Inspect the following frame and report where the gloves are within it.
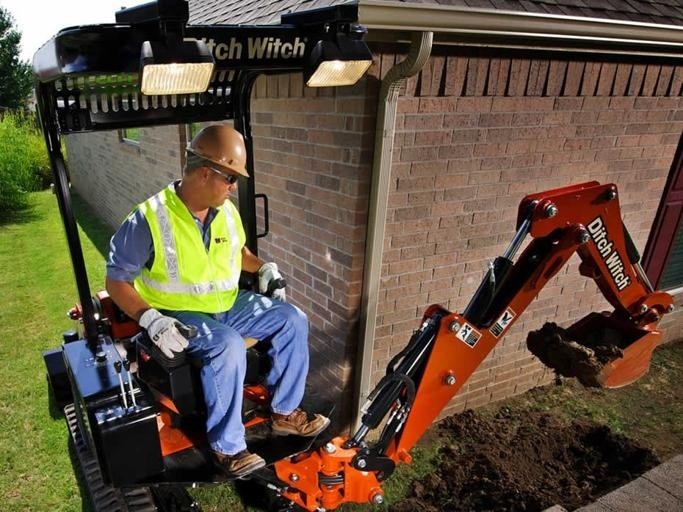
[139,308,190,359]
[258,263,286,303]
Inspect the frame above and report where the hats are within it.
[186,125,249,178]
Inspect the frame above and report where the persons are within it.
[104,124,331,477]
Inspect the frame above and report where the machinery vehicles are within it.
[25,2,677,511]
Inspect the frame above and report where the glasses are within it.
[210,165,237,184]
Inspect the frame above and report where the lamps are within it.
[113,1,373,97]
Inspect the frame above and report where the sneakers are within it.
[272,408,331,437]
[213,448,266,476]
